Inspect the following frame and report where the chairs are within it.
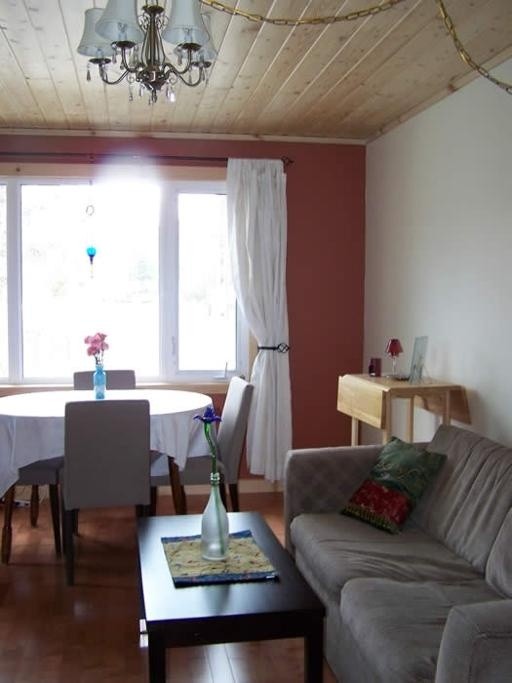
[15,465,61,554]
[72,370,144,536]
[150,376,254,516]
[63,400,150,587]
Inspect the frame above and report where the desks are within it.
[336,371,471,446]
[0,389,216,563]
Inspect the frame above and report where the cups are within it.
[369,358,381,376]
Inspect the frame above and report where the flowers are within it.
[84,332,109,366]
[193,407,225,556]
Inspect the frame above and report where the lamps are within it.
[77,0,219,103]
[385,338,404,380]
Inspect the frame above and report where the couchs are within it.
[284,424,512,683]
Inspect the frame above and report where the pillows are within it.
[339,436,447,535]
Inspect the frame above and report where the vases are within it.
[201,472,230,561]
[93,364,107,399]
